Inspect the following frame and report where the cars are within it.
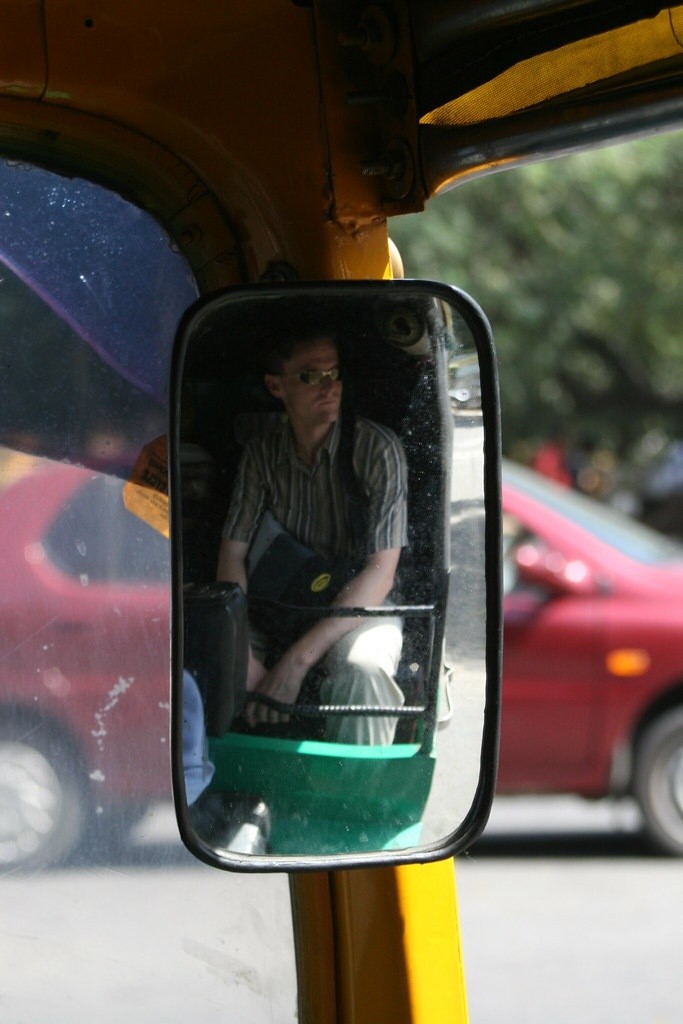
[0,445,683,872]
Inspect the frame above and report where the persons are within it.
[216,327,406,742]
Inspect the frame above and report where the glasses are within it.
[277,364,345,385]
